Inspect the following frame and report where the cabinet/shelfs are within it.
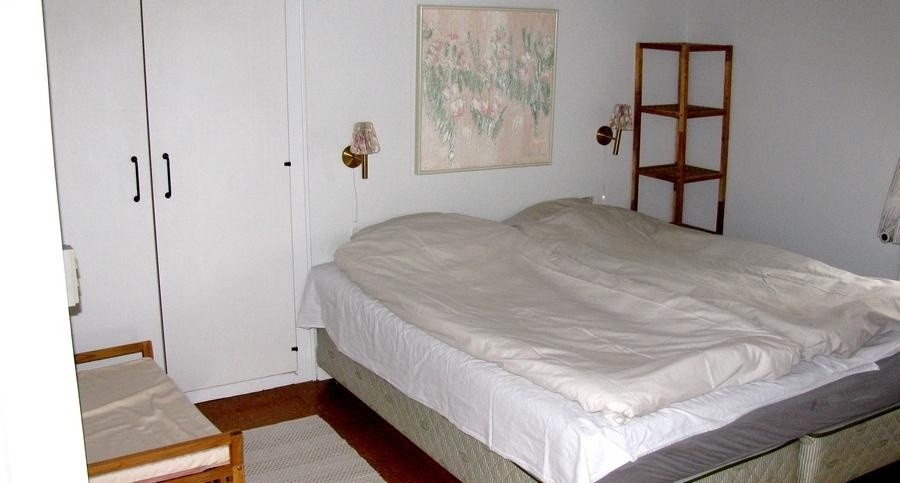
[631,41,733,234]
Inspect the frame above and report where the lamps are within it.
[342,120,379,179]
[596,104,632,155]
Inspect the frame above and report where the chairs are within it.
[74,341,245,483]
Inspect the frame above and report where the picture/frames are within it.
[416,3,559,176]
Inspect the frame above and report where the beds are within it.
[295,199,900,483]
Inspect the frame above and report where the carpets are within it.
[220,413,388,483]
